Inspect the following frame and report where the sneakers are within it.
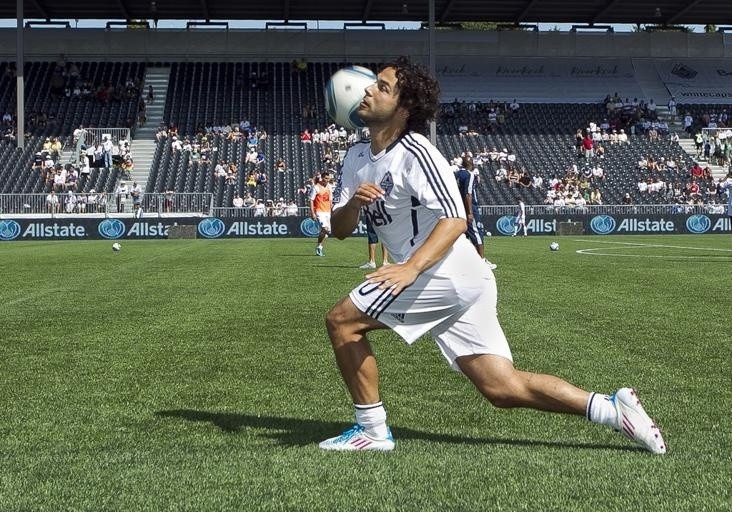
[360,261,377,269]
[316,245,324,255]
[318,425,397,451]
[614,387,667,455]
[483,258,498,271]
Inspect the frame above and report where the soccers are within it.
[485,231,491,237]
[549,241,559,252]
[112,243,121,251]
[322,64,379,132]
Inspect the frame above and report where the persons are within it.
[426,98,520,137]
[544,162,605,214]
[512,196,528,238]
[1,62,155,213]
[669,96,732,165]
[309,171,334,257]
[317,54,668,456]
[155,56,362,216]
[576,92,667,162]
[358,211,391,269]
[623,154,731,212]
[451,154,497,270]
[450,147,543,192]
